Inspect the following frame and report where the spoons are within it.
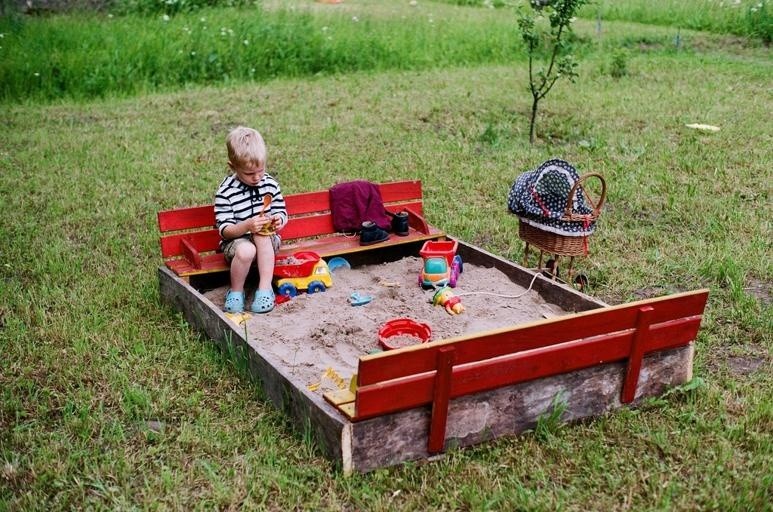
[259,194,272,218]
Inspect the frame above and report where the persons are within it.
[213,123,288,315]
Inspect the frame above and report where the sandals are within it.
[225,288,246,313]
[251,287,276,313]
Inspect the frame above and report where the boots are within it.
[360,220,389,244]
[392,212,409,236]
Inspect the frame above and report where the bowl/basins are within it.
[378,318,431,352]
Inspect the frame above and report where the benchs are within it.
[318,284,711,457]
[154,176,447,287]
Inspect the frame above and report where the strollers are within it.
[506,158,606,293]
